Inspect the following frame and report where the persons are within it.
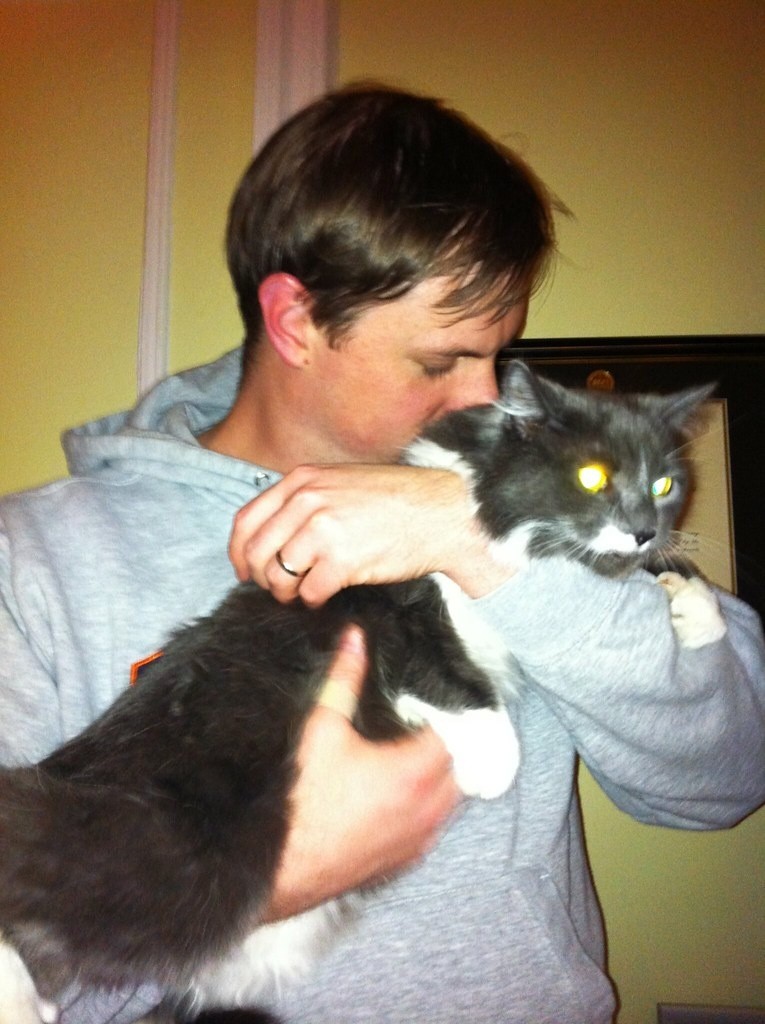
[0,82,765,1024]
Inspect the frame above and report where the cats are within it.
[0,358,719,1024]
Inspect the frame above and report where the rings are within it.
[317,680,359,721]
[276,550,306,578]
